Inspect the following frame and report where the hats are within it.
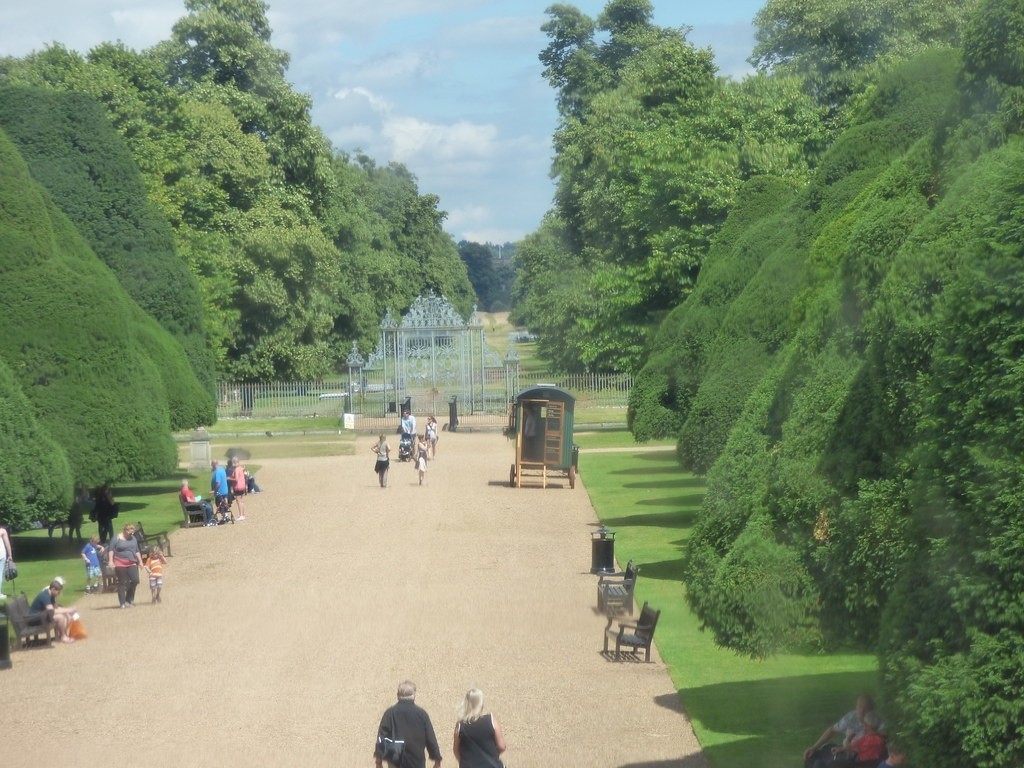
[54,577,66,586]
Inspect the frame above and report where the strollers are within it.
[399,433,415,462]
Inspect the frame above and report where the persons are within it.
[399,409,416,455]
[362,377,368,398]
[47,486,88,545]
[372,681,443,768]
[81,535,104,593]
[425,416,437,459]
[108,522,144,608]
[805,695,909,768]
[0,527,13,599]
[370,433,391,486]
[180,479,217,526]
[209,456,263,524]
[415,434,428,486]
[144,546,168,604]
[452,688,506,768]
[90,484,115,543]
[525,405,537,462]
[222,381,240,404]
[27,576,76,644]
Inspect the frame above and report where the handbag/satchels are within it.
[415,460,420,469]
[90,509,98,522]
[66,615,87,640]
[6,559,17,581]
[384,709,406,765]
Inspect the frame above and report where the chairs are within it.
[179,494,211,528]
[97,543,139,593]
[135,521,173,559]
[604,601,661,663]
[597,559,638,616]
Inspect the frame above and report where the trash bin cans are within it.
[589,525,617,575]
[563,446,579,473]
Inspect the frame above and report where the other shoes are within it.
[236,516,245,521]
[87,582,162,609]
[208,522,218,526]
[62,635,75,643]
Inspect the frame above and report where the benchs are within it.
[3,591,51,649]
